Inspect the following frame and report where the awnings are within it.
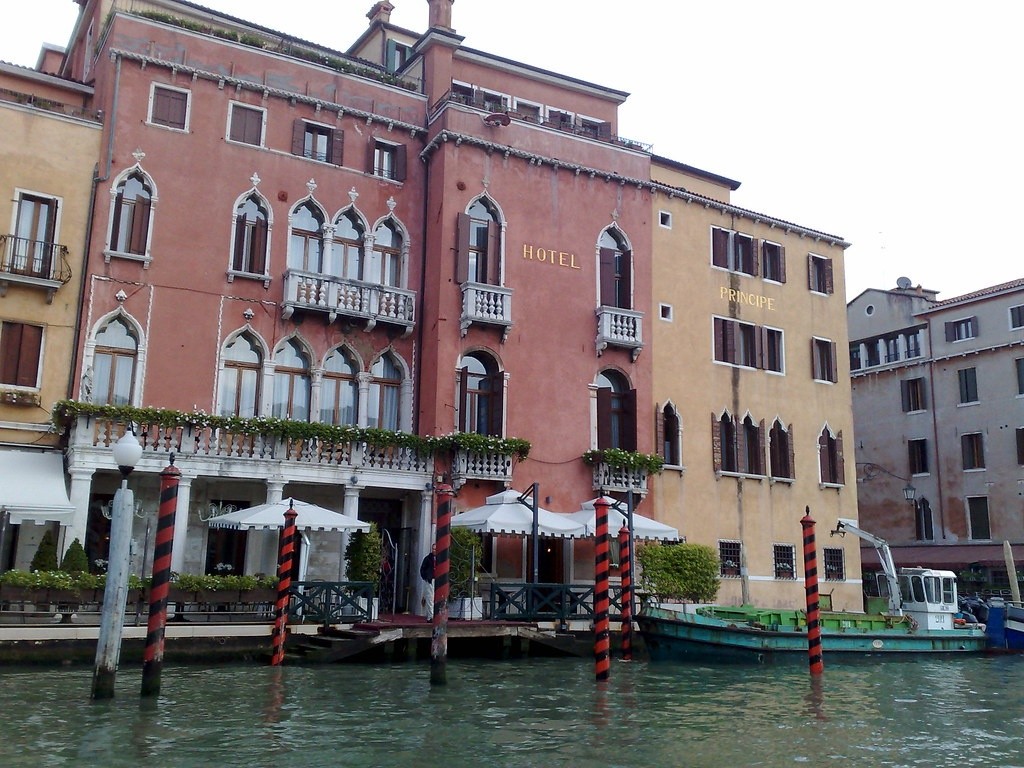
[0,449,73,528]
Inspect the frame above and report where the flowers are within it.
[582,448,666,477]
[452,92,468,101]
[145,572,199,593]
[439,430,532,463]
[723,560,738,568]
[0,569,50,590]
[50,571,98,591]
[48,399,192,436]
[97,574,144,591]
[359,425,439,459]
[239,576,280,592]
[777,563,792,572]
[193,403,281,441]
[282,414,360,446]
[130,9,418,90]
[826,564,838,575]
[199,573,241,592]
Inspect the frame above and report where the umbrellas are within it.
[208,478,680,617]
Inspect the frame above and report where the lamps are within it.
[902,483,916,507]
[100,499,148,519]
[197,501,243,531]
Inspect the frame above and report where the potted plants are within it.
[342,520,386,620]
[488,101,505,113]
[29,528,59,612]
[448,525,484,620]
[560,122,576,133]
[471,96,486,110]
[520,112,539,124]
[577,126,598,139]
[539,119,560,129]
[55,538,89,624]
[626,142,643,151]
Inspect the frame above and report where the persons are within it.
[957,592,989,624]
[420,543,436,622]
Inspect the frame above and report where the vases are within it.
[196,591,240,602]
[396,80,404,88]
[240,588,276,601]
[95,590,140,603]
[369,74,376,80]
[452,97,466,104]
[200,27,213,35]
[609,140,625,146]
[505,111,523,120]
[143,590,196,602]
[406,83,414,91]
[830,574,838,579]
[47,590,95,602]
[726,567,737,575]
[595,136,608,142]
[778,571,793,578]
[0,584,48,603]
